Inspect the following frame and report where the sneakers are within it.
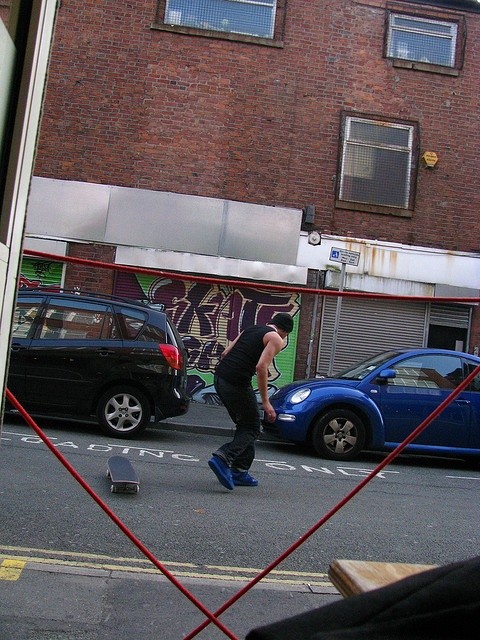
[208,454,235,490]
[230,468,259,486]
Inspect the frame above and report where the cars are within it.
[263,324,480,464]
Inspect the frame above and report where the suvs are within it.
[0,287,190,436]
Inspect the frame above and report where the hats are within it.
[273,312,294,333]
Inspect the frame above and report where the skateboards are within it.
[105,455,140,494]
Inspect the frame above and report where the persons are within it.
[208,313,294,490]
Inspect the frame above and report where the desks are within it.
[329,557,440,598]
